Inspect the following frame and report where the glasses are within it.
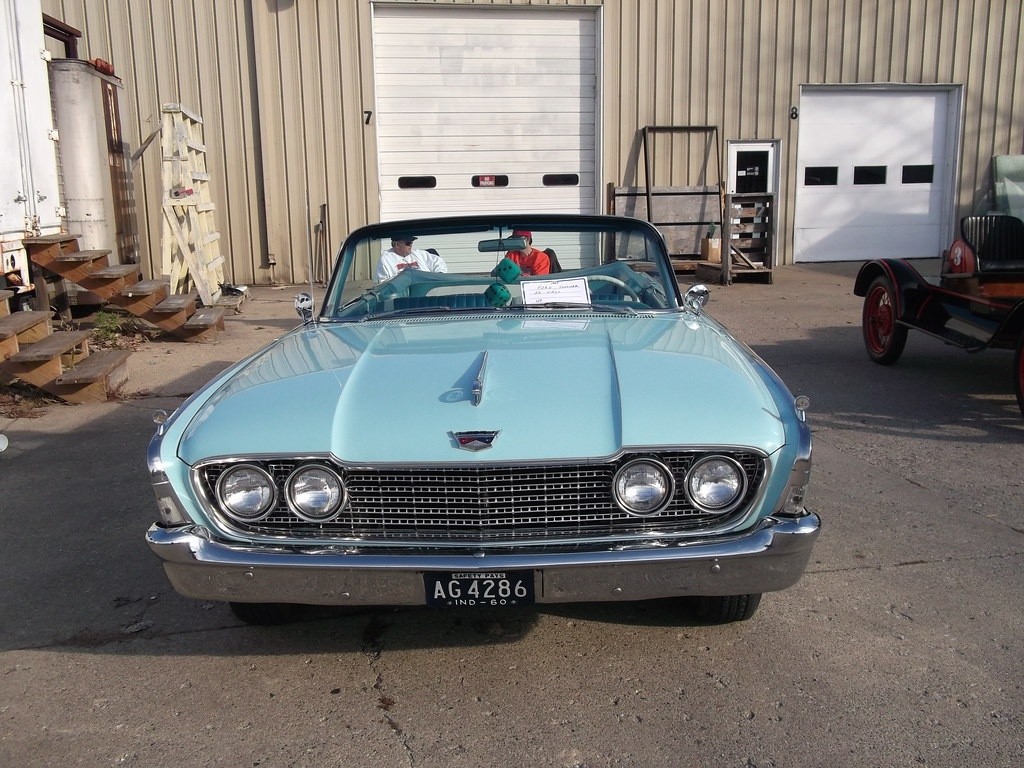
[398,241,413,246]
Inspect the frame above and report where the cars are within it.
[144,212,820,625]
[852,214,1024,417]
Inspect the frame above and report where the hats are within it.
[391,236,418,241]
[507,230,531,239]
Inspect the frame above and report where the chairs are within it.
[960,216,1024,299]
[384,293,491,312]
[590,300,652,310]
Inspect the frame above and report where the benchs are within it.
[409,279,624,308]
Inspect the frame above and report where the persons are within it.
[374,236,447,284]
[505,230,550,276]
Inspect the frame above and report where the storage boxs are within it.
[701,237,722,262]
